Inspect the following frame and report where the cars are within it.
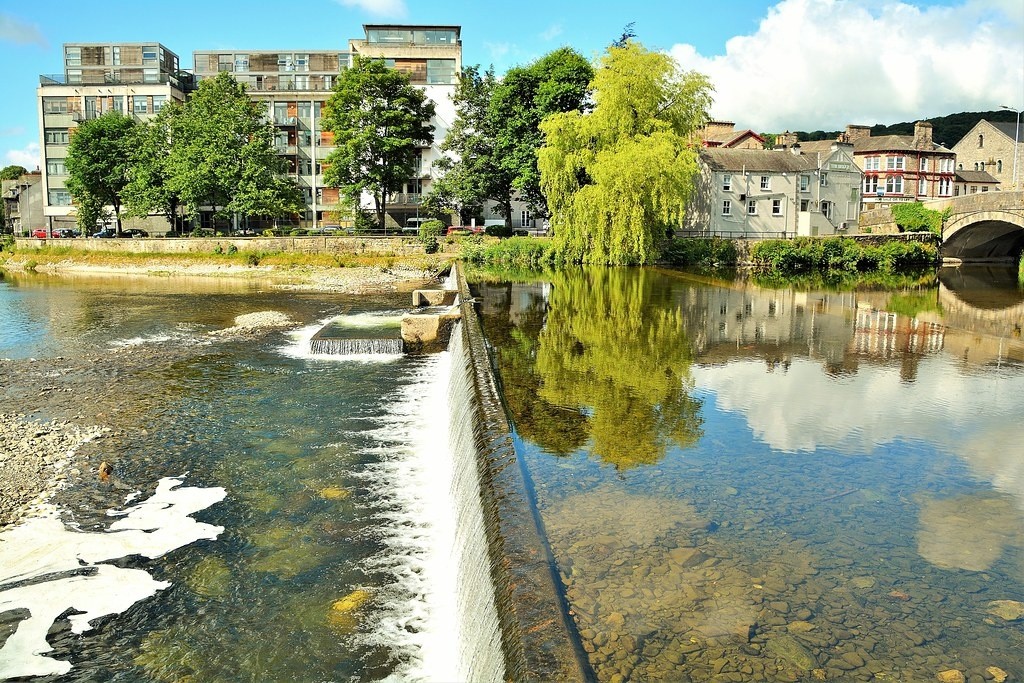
[448,226,479,234]
[32,229,82,239]
[165,231,180,237]
[118,228,149,238]
[92,228,116,238]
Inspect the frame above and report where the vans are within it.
[402,217,438,236]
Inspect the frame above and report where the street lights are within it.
[999,104,1021,186]
